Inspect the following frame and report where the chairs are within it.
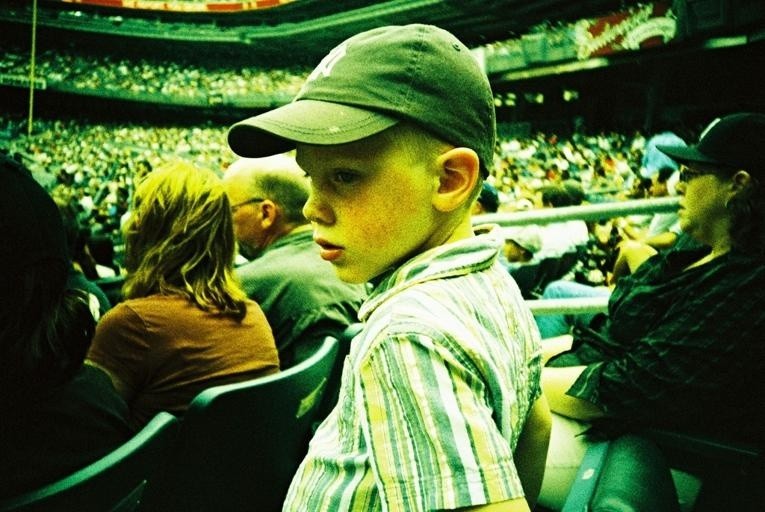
[1,243,683,510]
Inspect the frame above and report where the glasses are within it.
[677,166,721,184]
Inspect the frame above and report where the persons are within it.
[1,8,764,512]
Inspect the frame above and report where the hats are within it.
[653,110,765,168]
[224,21,500,182]
